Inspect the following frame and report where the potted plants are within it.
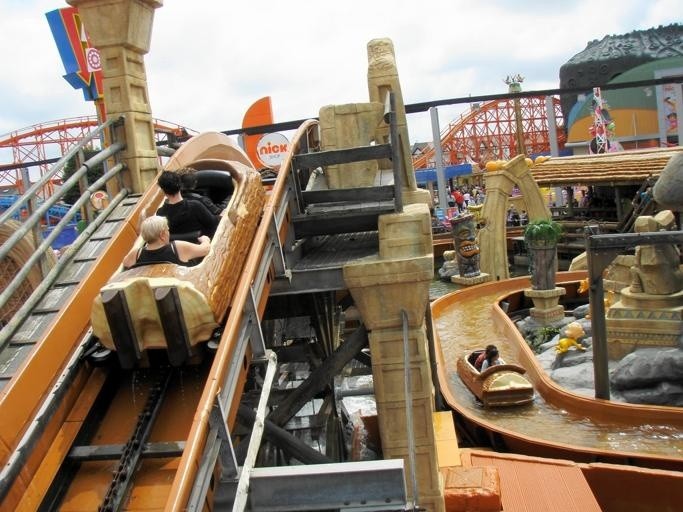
[523,215,563,291]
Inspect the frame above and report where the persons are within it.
[562,186,656,222]
[505,204,528,226]
[429,182,486,234]
[123,166,231,271]
[663,97,677,132]
[472,344,504,374]
